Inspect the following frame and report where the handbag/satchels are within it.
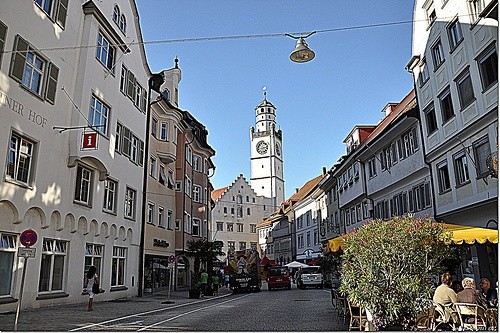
[92,276,100,294]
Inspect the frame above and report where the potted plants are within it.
[186,240,225,299]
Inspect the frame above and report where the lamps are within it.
[285,31,317,63]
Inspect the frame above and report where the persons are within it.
[225,273,229,289]
[432,272,499,310]
[212,274,219,296]
[86,266,99,312]
[201,269,208,295]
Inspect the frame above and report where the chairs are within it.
[332,289,499,332]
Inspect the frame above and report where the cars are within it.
[295,264,323,290]
[266,266,293,291]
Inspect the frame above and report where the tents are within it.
[327,220,499,252]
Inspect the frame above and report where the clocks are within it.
[257,143,268,153]
